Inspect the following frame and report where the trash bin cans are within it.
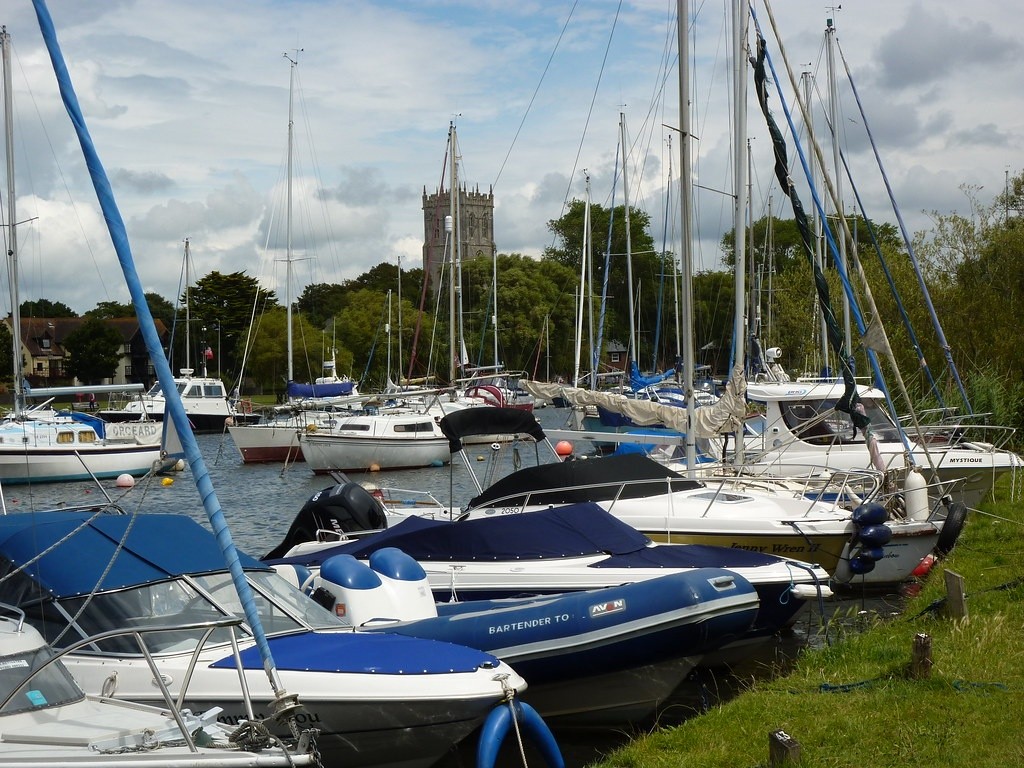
[75,393,85,402]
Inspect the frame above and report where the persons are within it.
[109,392,117,408]
[233,386,241,399]
[88,392,96,411]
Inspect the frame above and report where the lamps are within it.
[766,347,782,362]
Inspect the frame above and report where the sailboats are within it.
[0,0,1024,768]
